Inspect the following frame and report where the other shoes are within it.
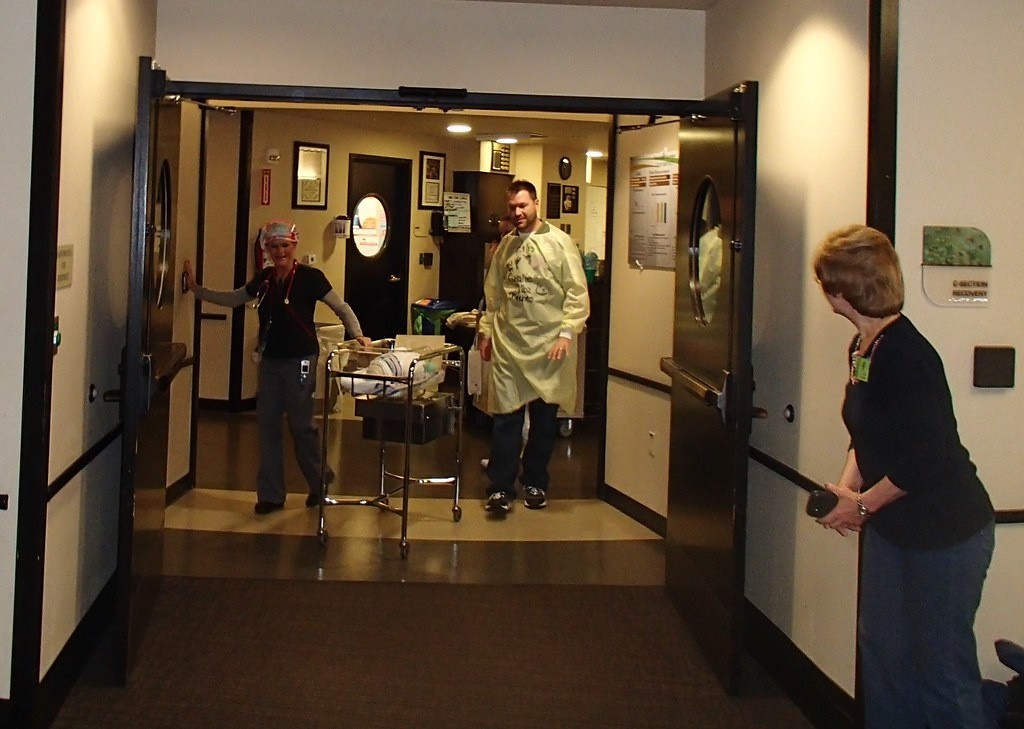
[480,459,489,468]
[255,501,284,514]
[520,444,527,460]
[305,473,335,507]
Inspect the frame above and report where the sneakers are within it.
[485,490,514,510]
[522,485,546,507]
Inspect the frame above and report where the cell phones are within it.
[806,490,838,518]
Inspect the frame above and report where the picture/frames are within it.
[418,151,446,210]
[292,140,327,207]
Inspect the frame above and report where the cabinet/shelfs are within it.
[452,170,514,242]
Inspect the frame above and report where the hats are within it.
[260,219,299,250]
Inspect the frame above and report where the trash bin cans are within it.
[411,298,461,386]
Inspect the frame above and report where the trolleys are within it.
[446,295,574,439]
[316,342,466,562]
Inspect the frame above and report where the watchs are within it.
[856,494,873,518]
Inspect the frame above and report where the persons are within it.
[695,206,723,323]
[184,219,372,513]
[811,227,997,729]
[477,179,591,514]
